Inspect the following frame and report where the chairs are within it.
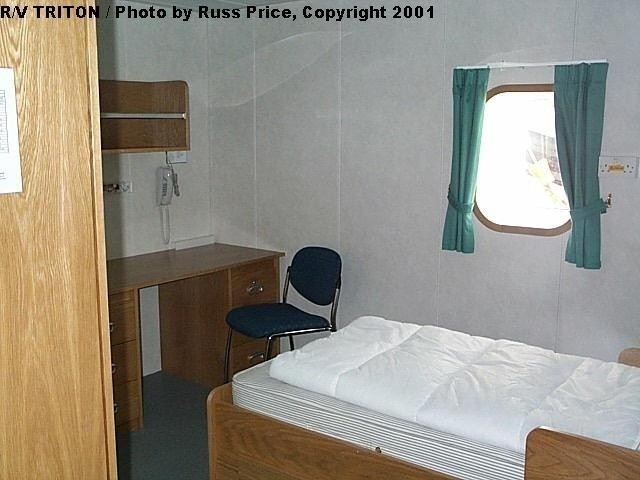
[224,246,343,384]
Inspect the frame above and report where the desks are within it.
[106,240,286,438]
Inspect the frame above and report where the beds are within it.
[206,315,640,480]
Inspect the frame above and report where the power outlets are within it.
[166,151,188,164]
[597,156,639,178]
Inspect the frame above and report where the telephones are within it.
[156,166,174,206]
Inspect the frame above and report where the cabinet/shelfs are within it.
[99,79,190,155]
[0,2,118,480]
